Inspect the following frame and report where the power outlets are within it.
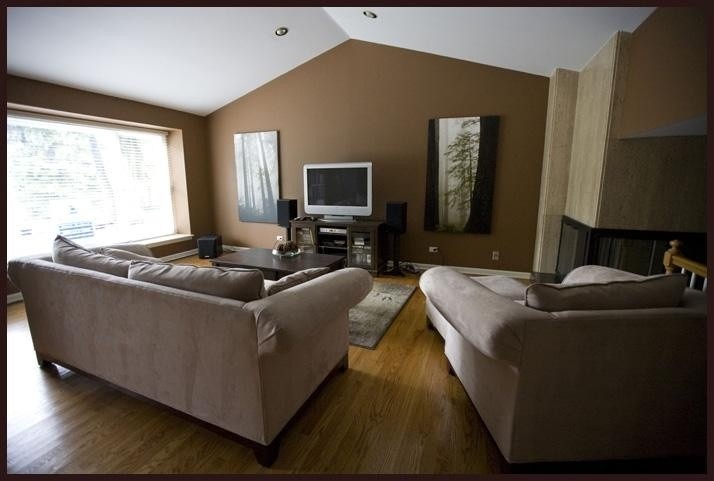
[428,245,440,254]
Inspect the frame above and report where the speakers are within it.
[277,199,297,227]
[386,202,407,235]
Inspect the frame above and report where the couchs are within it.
[8,241,374,468]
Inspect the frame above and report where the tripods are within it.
[384,235,405,277]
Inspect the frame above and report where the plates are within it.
[272,249,301,258]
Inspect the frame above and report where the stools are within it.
[421,270,526,332]
[196,233,224,257]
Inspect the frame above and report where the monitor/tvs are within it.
[303,162,373,222]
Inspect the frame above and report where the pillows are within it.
[49,233,133,278]
[266,264,330,299]
[126,259,263,301]
[523,271,689,314]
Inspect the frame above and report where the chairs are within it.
[417,258,709,470]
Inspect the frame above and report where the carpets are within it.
[345,278,418,349]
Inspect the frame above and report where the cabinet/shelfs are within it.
[287,217,387,277]
